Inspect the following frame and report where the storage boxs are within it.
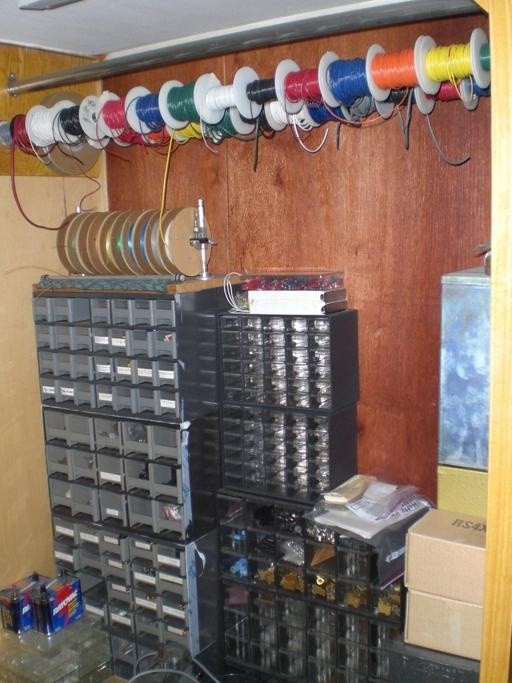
[437,266,491,472]
[437,464,488,520]
[404,589,484,661]
[404,507,487,606]
